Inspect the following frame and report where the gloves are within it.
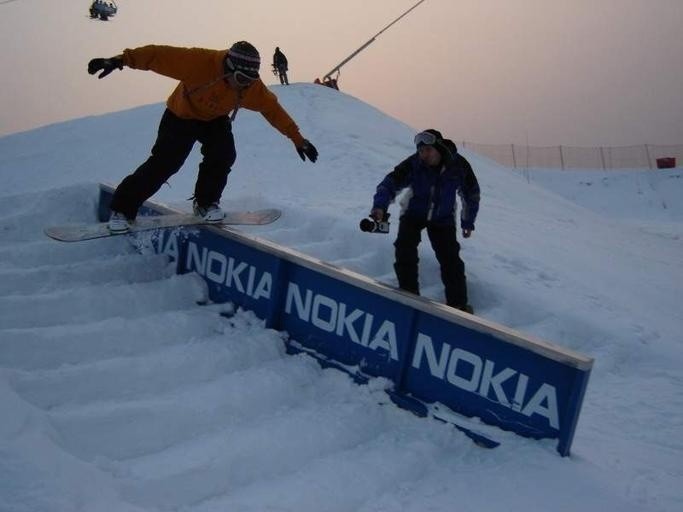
[296,139,318,162]
[88,56,124,79]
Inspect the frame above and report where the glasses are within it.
[225,57,260,88]
[415,132,443,145]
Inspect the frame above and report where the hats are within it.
[416,130,443,154]
[225,41,260,78]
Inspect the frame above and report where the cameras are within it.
[360,212,390,233]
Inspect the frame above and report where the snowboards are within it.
[44,209,281,242]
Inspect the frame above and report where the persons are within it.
[370,128,479,312]
[89,0,118,20]
[270,44,288,87]
[83,41,320,235]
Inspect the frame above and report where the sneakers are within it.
[108,210,129,234]
[193,198,224,220]
